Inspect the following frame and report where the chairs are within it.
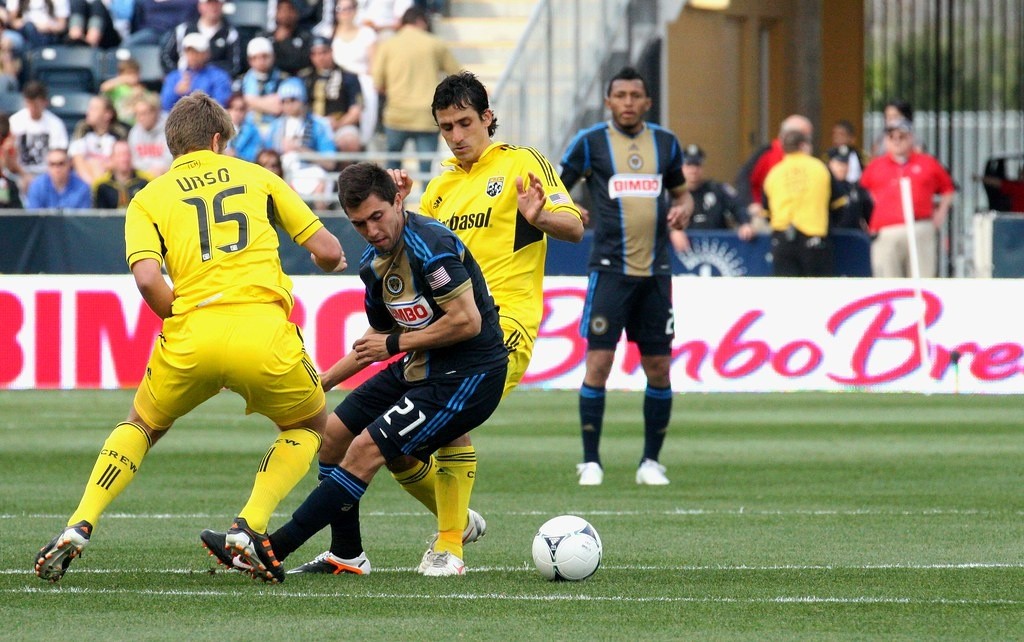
[2,42,173,151]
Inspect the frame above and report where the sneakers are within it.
[226,517,285,584]
[636,465,670,486]
[33,519,93,584]
[201,529,234,570]
[416,508,487,574]
[578,462,603,485]
[424,552,467,575]
[301,552,371,575]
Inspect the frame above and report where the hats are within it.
[278,76,309,104]
[678,149,705,166]
[246,37,272,55]
[182,32,210,52]
[827,146,858,162]
[308,37,330,47]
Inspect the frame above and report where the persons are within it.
[667,97,957,278]
[0,0,468,208]
[382,66,585,579]
[198,161,512,584]
[34,91,349,585]
[557,64,696,487]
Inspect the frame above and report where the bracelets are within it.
[385,332,403,357]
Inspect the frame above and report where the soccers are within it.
[531,513,603,582]
